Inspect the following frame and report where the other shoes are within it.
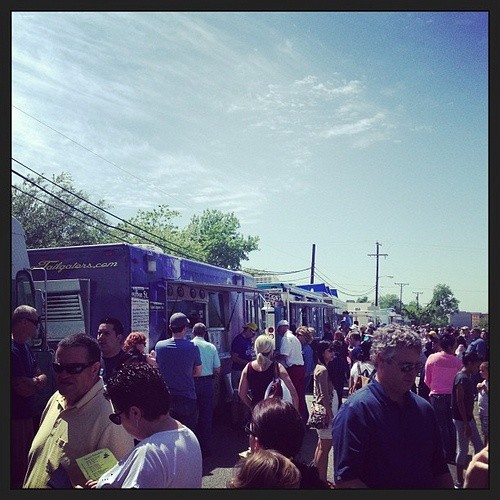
[455,480,465,489]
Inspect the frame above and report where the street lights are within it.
[375,276,394,306]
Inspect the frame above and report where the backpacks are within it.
[348,362,377,396]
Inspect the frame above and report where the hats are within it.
[276,320,288,331]
[242,323,259,331]
[429,331,436,337]
[170,312,190,328]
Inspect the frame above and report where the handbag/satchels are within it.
[269,362,283,401]
[308,402,330,429]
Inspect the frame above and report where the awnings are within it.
[289,301,342,324]
[166,279,269,330]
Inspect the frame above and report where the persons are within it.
[190,322,221,432]
[424,333,461,464]
[275,320,304,417]
[231,322,258,402]
[155,312,202,433]
[452,351,484,489]
[331,323,455,488]
[244,398,331,489]
[226,449,301,489]
[323,311,487,410]
[84,360,203,489]
[22,333,134,488]
[476,362,489,445]
[237,334,299,410]
[122,332,157,369]
[296,326,315,395]
[97,318,133,386]
[10,305,48,489]
[313,341,338,488]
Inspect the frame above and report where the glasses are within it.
[244,423,255,437]
[251,330,256,335]
[23,318,38,326]
[52,363,93,375]
[389,358,420,372]
[109,410,125,425]
[140,342,146,346]
[327,348,336,353]
